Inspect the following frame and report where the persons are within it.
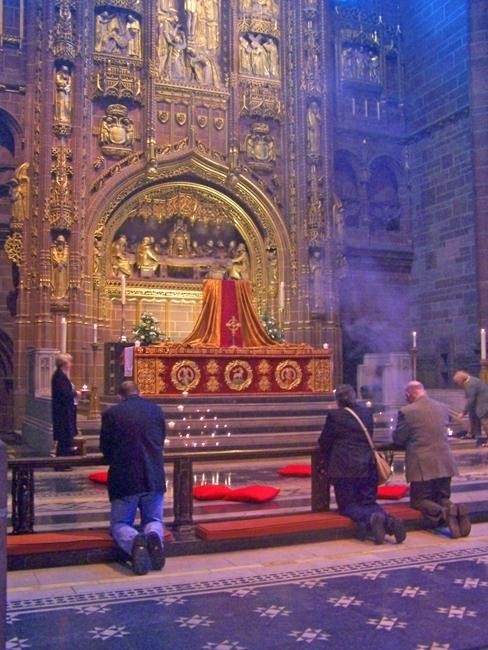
[317,383,407,545]
[98,380,168,576]
[51,234,69,299]
[110,235,135,279]
[392,379,472,539]
[136,236,161,271]
[229,243,250,280]
[50,352,83,472]
[54,65,72,122]
[453,369,488,447]
[168,218,192,258]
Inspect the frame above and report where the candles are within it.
[120,275,126,304]
[92,324,98,343]
[278,282,285,308]
[479,329,486,360]
[58,318,67,354]
[411,331,417,348]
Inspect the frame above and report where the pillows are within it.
[277,462,312,477]
[377,484,409,499]
[227,480,279,503]
[193,484,233,500]
[87,470,108,484]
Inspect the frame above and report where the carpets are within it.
[2,538,487,650]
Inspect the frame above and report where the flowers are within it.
[261,312,286,343]
[130,310,168,344]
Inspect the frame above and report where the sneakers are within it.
[372,512,386,545]
[457,503,471,537]
[147,531,165,571]
[131,533,149,576]
[443,502,462,538]
[391,516,406,543]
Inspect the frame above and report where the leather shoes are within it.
[460,434,475,439]
[482,442,487,447]
[55,466,73,471]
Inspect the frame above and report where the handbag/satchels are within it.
[375,457,393,485]
